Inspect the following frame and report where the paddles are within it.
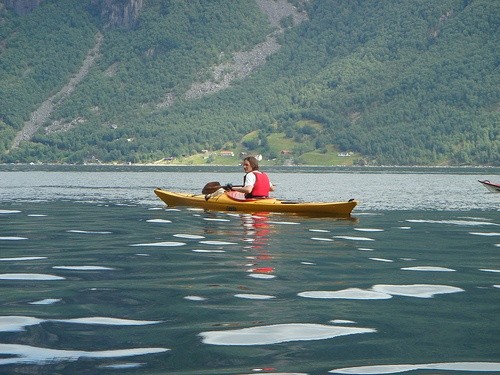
[202,181,276,195]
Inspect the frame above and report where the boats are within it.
[477,179,500,192]
[153,189,357,215]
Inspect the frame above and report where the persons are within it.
[224,156,275,199]
